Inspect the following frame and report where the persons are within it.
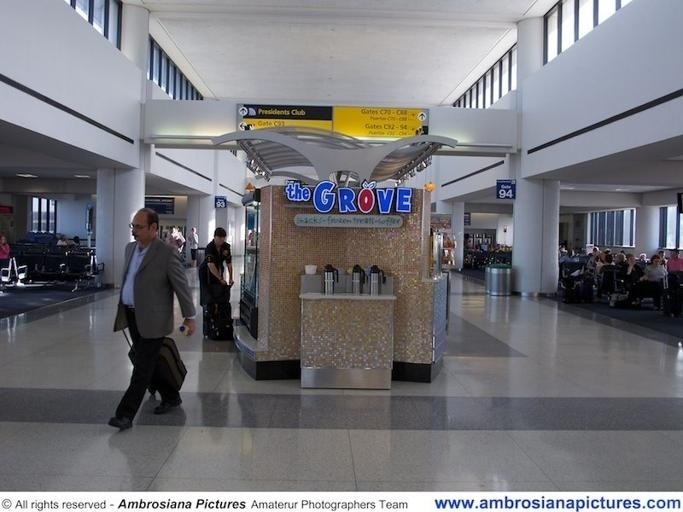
[197,228,233,338]
[68,236,79,247]
[165,226,198,268]
[56,234,68,246]
[104,206,196,430]
[464,238,683,313]
[1,234,10,259]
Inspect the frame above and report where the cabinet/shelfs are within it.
[298,292,396,391]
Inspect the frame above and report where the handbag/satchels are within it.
[200,279,236,342]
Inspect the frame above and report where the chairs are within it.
[559,244,683,316]
[0,231,97,293]
[463,243,512,268]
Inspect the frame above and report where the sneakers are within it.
[151,394,184,416]
[107,413,134,430]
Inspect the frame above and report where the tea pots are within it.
[370,264,387,295]
[324,263,338,295]
[351,264,366,295]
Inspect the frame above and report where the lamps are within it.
[394,142,441,189]
[239,142,272,182]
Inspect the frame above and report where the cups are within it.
[304,264,317,275]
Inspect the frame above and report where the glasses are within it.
[128,223,153,231]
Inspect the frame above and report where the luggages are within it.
[123,334,188,396]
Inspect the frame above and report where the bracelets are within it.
[218,278,222,281]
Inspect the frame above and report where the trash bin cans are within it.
[485,264,512,296]
[196,248,206,267]
